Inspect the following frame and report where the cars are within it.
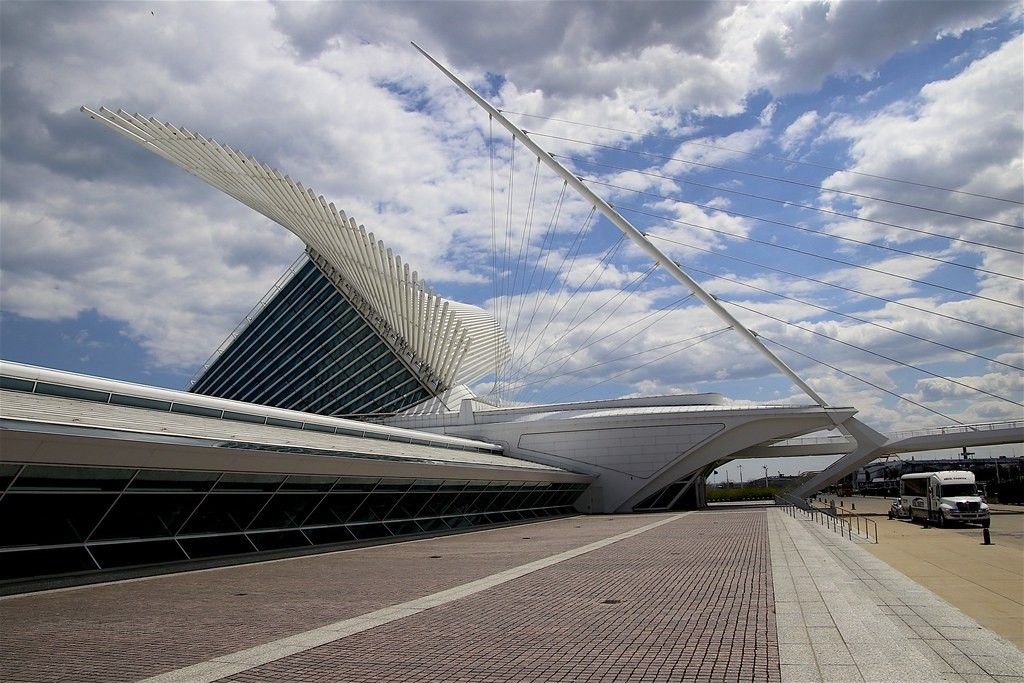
[890,498,908,519]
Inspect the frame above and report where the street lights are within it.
[737,464,744,488]
[762,465,769,487]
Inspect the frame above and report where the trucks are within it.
[901,471,991,529]
